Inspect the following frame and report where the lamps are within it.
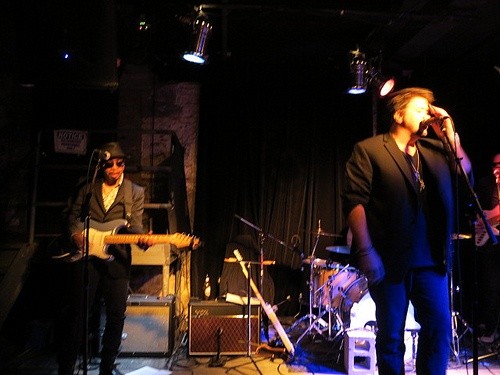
[182,4,214,64]
[347,44,397,98]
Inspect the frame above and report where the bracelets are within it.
[355,245,374,259]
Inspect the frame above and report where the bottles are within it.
[202,274,211,301]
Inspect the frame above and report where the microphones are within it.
[292,243,305,259]
[419,116,446,132]
[95,148,111,161]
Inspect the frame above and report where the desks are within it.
[128,240,181,322]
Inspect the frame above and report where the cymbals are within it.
[325,245,351,254]
[449,232,473,240]
[304,229,344,238]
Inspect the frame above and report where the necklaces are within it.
[397,144,425,192]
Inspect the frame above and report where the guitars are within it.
[58,215,203,266]
[475,204,500,246]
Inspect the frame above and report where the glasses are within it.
[103,159,125,168]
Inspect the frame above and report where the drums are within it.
[310,263,358,306]
[299,257,341,308]
[340,275,420,365]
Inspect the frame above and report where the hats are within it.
[101,141,125,161]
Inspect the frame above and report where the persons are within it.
[59,142,153,375]
[340,88,471,375]
[471,152,500,351]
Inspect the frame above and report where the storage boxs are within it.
[189,297,262,356]
[100,294,175,357]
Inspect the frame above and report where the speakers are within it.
[188,299,261,355]
[98,293,175,357]
